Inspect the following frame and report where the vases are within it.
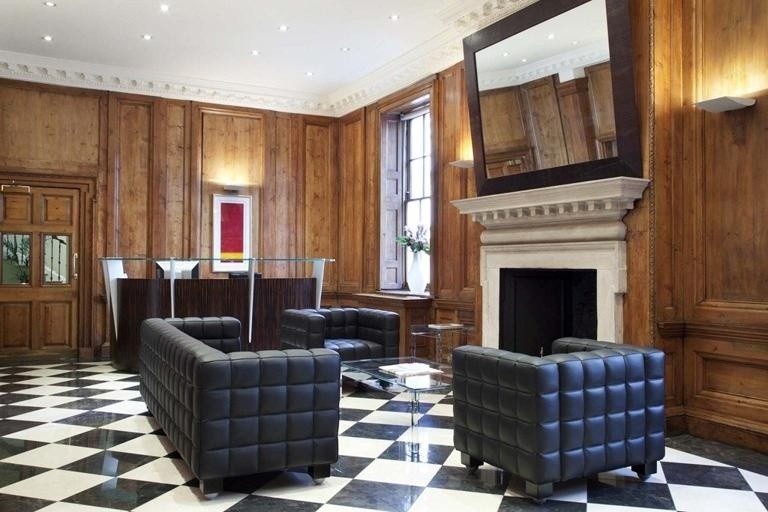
[405,251,427,293]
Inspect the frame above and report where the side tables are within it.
[409,323,470,386]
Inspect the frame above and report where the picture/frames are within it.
[211,193,253,273]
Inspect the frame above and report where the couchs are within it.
[137,315,340,500]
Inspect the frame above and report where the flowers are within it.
[394,225,430,256]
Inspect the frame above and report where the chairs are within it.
[452,336,666,505]
[276,305,400,391]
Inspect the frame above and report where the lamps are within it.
[692,95,757,115]
[223,185,241,193]
[449,159,475,170]
